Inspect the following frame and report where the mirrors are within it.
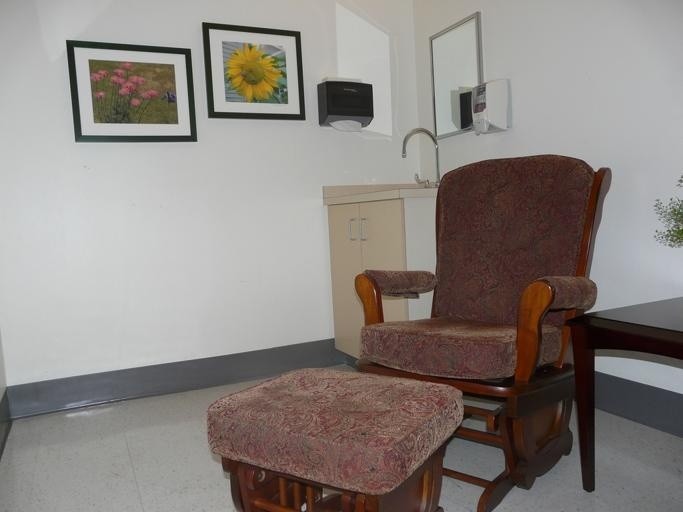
[427,12,484,142]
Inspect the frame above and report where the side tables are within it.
[563,295,681,492]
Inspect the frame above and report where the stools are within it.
[205,365,464,511]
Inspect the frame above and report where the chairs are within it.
[354,154,612,512]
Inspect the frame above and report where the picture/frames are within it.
[64,19,307,144]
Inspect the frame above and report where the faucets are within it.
[401,127,440,188]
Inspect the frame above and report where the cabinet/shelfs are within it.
[327,199,437,361]
